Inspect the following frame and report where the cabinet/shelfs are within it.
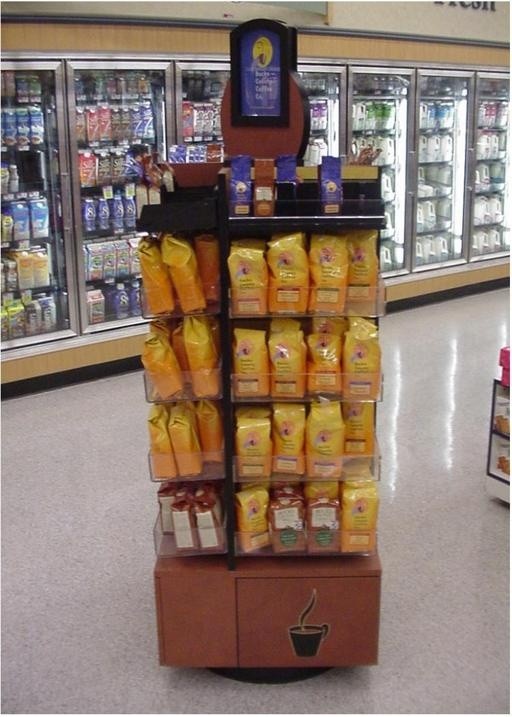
[140,162,382,684]
[483,378,510,504]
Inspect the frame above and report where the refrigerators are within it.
[470,70,512,263]
[343,62,416,277]
[74,62,174,335]
[0,58,81,357]
[413,66,475,272]
[292,64,348,168]
[170,62,235,165]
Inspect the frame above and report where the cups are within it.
[288,621,329,656]
